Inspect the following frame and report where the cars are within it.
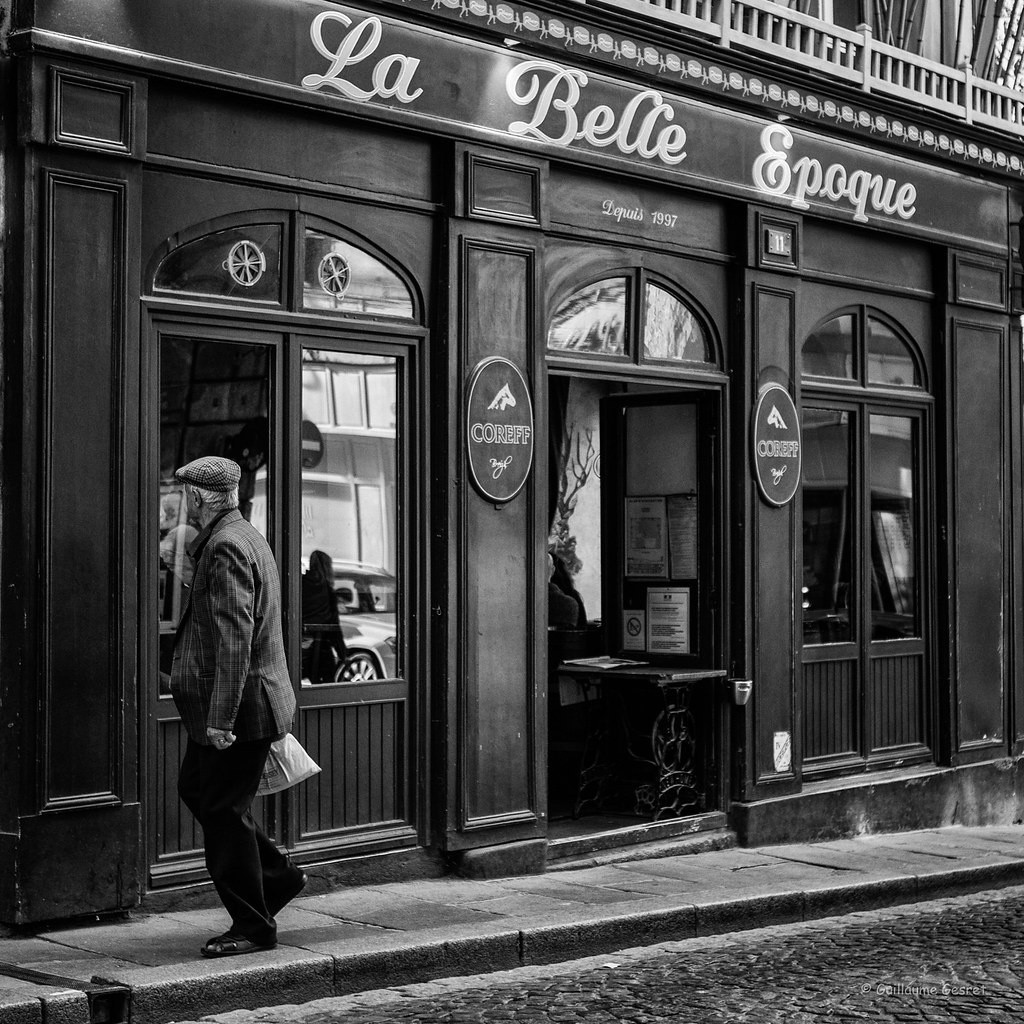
[299,559,398,686]
[802,606,914,644]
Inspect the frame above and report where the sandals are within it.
[199,930,277,954]
[265,864,306,916]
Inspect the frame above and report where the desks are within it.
[556,658,726,825]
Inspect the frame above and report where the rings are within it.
[219,739,225,742]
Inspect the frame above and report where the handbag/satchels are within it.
[255,731,322,796]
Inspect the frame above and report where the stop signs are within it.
[300,420,323,468]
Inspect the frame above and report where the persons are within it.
[547,551,588,625]
[303,549,350,685]
[160,493,201,620]
[174,456,309,956]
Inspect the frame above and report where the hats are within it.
[174,455,241,492]
